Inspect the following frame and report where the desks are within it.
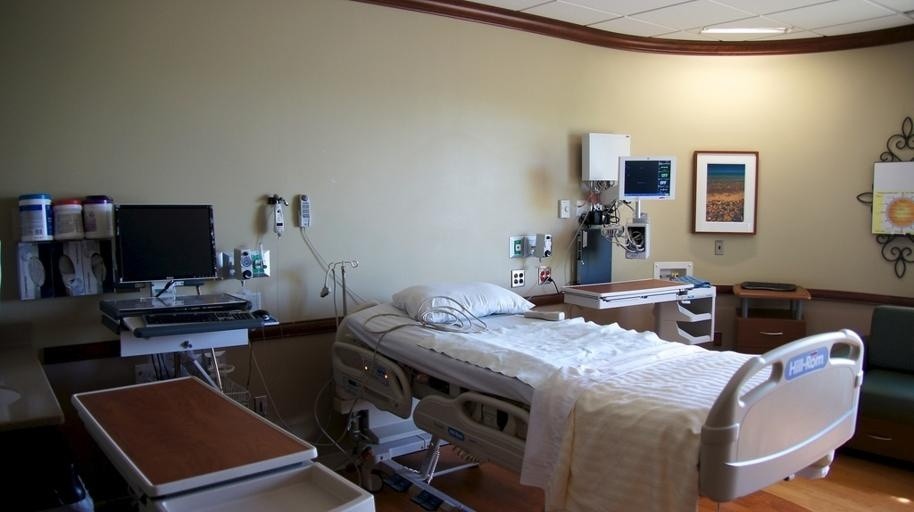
[553,272,695,313]
[93,292,277,397]
[66,371,378,512]
[0,298,348,512]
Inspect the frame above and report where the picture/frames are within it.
[687,146,760,239]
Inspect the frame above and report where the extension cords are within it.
[297,194,311,228]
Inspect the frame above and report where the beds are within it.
[322,270,873,512]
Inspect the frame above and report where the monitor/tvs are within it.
[113,204,218,298]
[619,156,677,200]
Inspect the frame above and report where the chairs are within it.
[833,299,913,474]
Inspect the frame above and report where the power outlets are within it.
[507,235,553,289]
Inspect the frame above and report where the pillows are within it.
[389,274,537,335]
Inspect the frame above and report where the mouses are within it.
[253,310,271,320]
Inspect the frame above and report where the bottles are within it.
[54,198,84,240]
[85,194,112,240]
[19,194,51,242]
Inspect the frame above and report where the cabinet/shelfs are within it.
[654,280,719,347]
[729,275,812,358]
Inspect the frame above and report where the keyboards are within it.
[143,312,256,328]
